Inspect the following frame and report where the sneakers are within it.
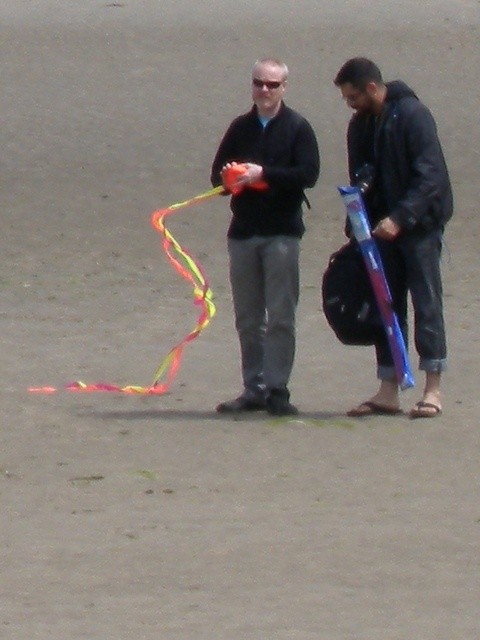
[265,392,298,415]
[217,395,264,412]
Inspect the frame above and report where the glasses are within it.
[342,90,365,101]
[252,79,285,90]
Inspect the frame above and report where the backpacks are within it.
[322,239,384,345]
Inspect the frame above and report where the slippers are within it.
[410,401,442,418]
[347,400,404,419]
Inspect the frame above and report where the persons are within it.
[210,57,321,415]
[324,57,454,419]
